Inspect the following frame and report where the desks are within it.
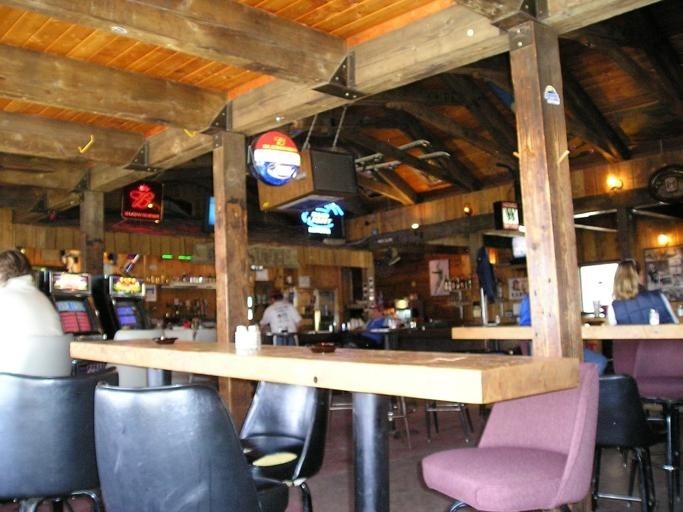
[69,325,582,511]
[451,327,681,376]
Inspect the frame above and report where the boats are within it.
[256,150,359,213]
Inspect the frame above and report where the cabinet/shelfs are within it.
[444,274,473,291]
[471,300,483,327]
[234,325,262,353]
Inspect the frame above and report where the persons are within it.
[606,256,681,327]
[648,260,664,290]
[0,250,72,379]
[255,289,299,347]
[344,305,403,350]
[517,279,530,326]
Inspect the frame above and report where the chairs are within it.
[95,379,290,510]
[2,369,118,512]
[421,363,599,507]
[236,380,325,512]
[584,338,682,508]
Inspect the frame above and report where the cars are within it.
[593,299,600,319]
[649,307,661,328]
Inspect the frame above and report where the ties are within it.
[115,300,146,331]
[505,221,526,265]
[55,298,96,334]
[292,202,347,242]
[201,194,215,235]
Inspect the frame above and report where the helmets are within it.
[309,342,337,353]
[151,336,178,345]
